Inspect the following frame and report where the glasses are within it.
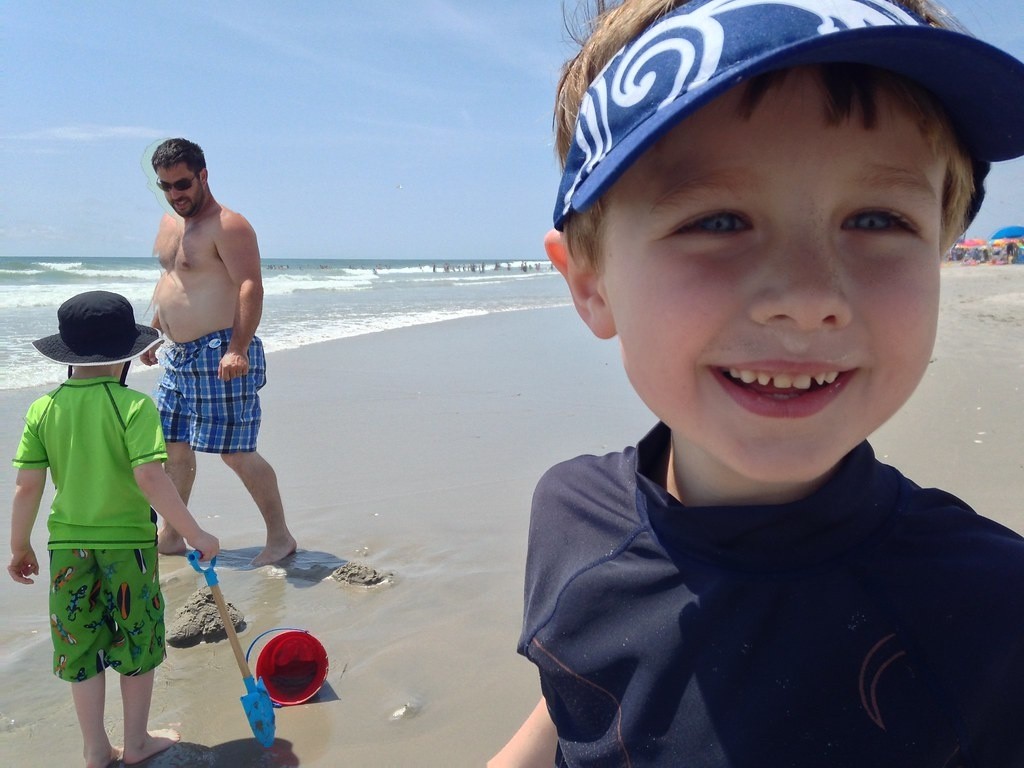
[156,174,199,191]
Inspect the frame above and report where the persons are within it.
[488,2,1023,768]
[139,138,297,567]
[5,291,221,767]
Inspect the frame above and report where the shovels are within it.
[188,550,276,746]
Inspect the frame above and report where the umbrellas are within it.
[955,226,1024,249]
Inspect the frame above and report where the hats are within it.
[32,291,164,366]
[554,0,1024,231]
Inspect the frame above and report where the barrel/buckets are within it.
[246,627,329,708]
